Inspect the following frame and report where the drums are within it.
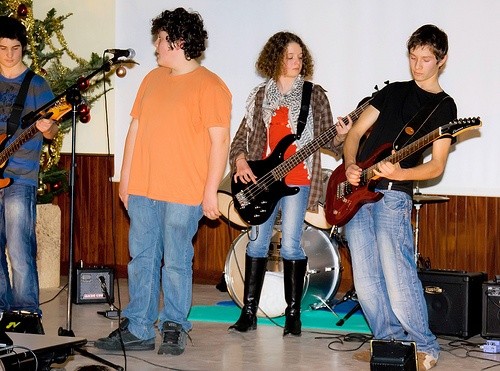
[221,224,343,317]
[216,171,254,228]
[304,168,333,230]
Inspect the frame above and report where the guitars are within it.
[0,93,85,190]
[216,80,391,230]
[323,116,482,227]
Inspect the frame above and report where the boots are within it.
[228,254,269,334]
[282,255,308,337]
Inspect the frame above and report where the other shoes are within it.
[352,349,371,362]
[417,351,439,371]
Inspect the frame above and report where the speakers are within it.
[416,269,499,340]
[71,268,115,304]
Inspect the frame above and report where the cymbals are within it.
[411,194,450,205]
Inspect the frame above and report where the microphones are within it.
[105,48,135,59]
[98,276,106,286]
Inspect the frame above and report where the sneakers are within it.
[0,311,45,334]
[158,321,192,355]
[94,318,155,350]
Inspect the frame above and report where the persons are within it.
[0,17,61,320]
[228,31,353,337]
[343,24,456,371]
[92,8,232,357]
